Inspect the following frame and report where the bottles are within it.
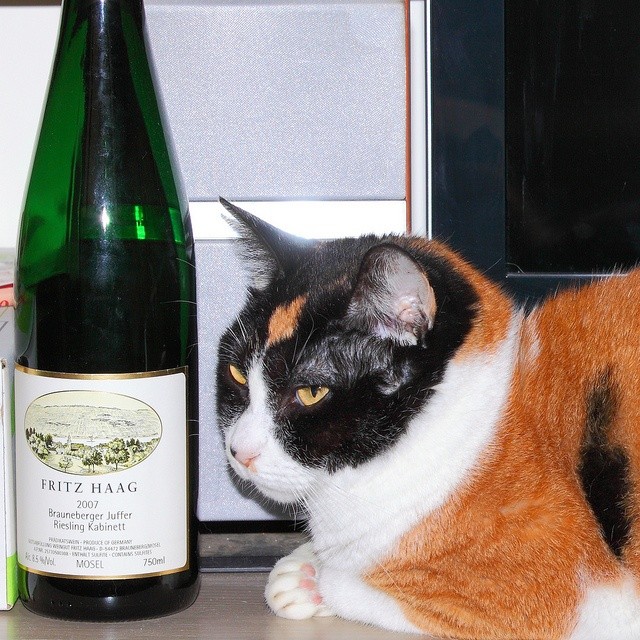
[14,0,200,623]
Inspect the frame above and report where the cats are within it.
[178,193,640,640]
